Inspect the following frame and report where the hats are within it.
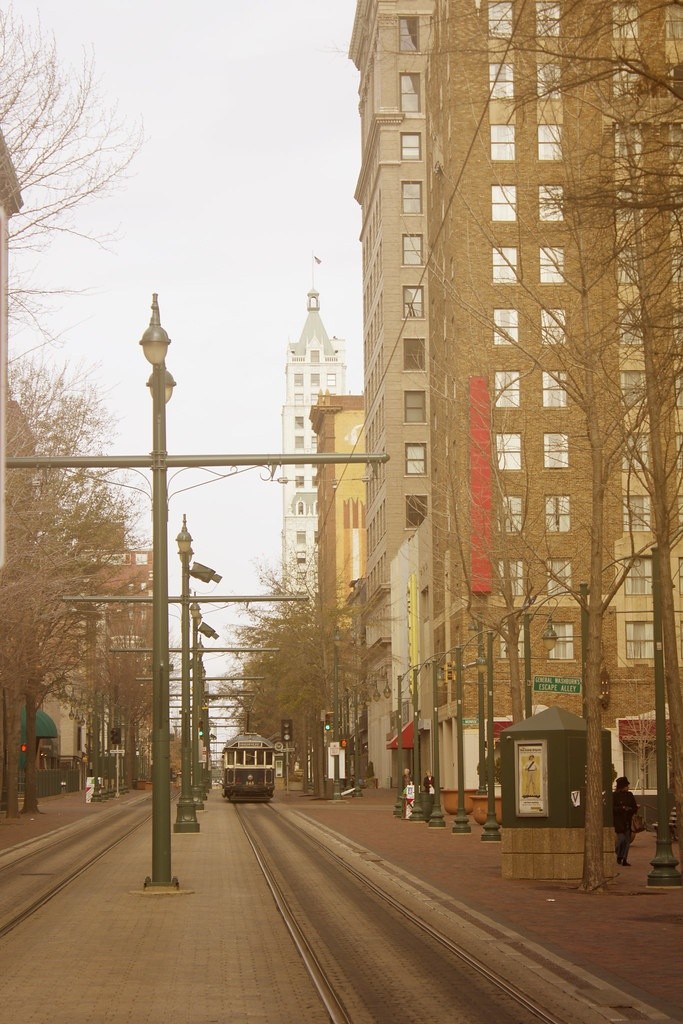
[616,777,630,790]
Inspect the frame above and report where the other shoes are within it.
[622,862,632,866]
[617,857,621,864]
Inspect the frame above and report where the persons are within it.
[423,769,435,793]
[612,776,638,866]
[350,775,355,788]
[402,768,413,792]
[523,755,538,795]
[343,776,348,787]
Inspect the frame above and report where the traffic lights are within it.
[281,719,293,742]
[340,739,347,747]
[324,713,330,731]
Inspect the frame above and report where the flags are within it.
[314,257,321,264]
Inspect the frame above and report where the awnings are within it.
[485,721,513,742]
[386,721,414,750]
[35,710,58,738]
[619,720,671,742]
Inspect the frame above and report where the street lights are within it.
[523,582,595,716]
[138,291,183,893]
[454,642,487,836]
[483,612,559,837]
[333,624,343,801]
[342,678,392,804]
[188,590,213,810]
[174,511,201,836]
[393,643,463,828]
[581,554,675,725]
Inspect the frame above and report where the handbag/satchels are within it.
[429,784,434,794]
[630,811,645,832]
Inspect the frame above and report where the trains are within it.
[222,731,276,804]
